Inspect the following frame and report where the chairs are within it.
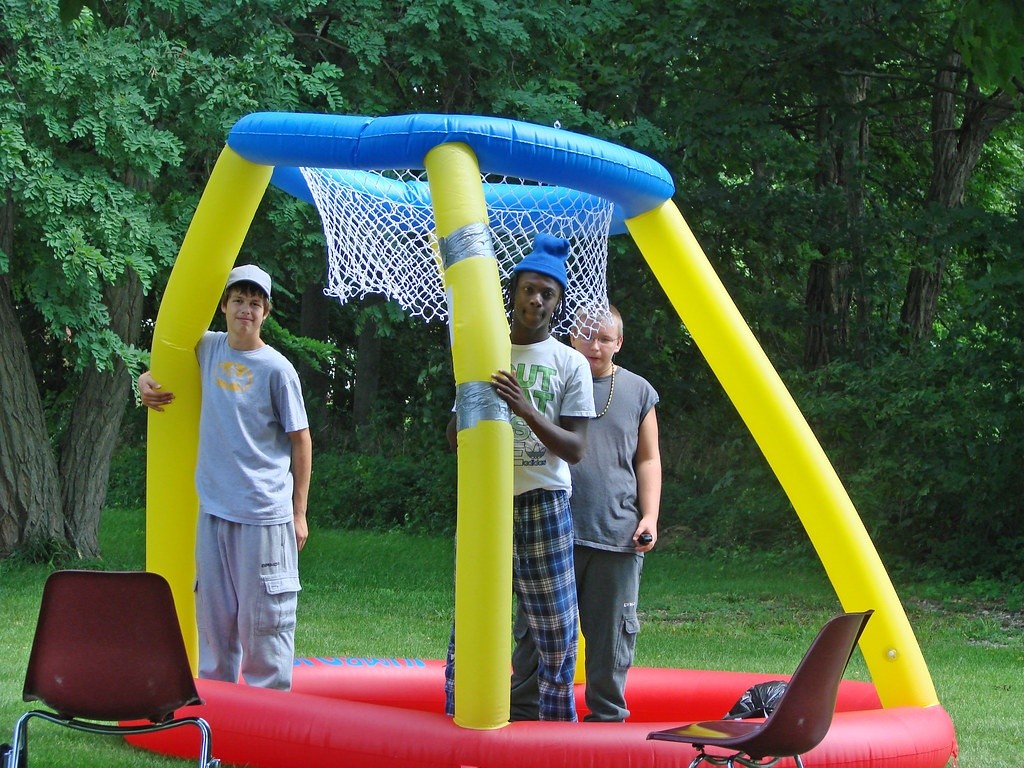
[6,568,222,768]
[648,609,877,768]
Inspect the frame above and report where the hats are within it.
[226,265,271,301]
[513,233,570,290]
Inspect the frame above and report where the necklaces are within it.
[591,362,614,418]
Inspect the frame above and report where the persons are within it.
[139,265,312,691]
[507,304,662,722]
[446,237,595,724]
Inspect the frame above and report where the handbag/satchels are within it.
[725,680,788,731]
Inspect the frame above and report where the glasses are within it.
[577,334,620,344]
